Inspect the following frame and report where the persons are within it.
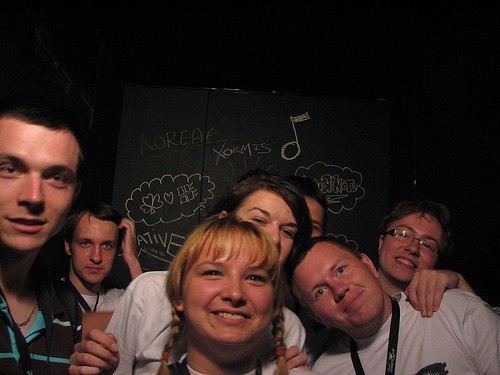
[68,176,475,375]
[158,211,314,375]
[0,113,83,375]
[288,236,500,375]
[65,205,142,312]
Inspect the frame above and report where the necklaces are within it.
[17,303,37,327]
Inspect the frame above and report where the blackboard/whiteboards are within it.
[110,82,392,286]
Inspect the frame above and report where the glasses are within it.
[384,228,441,256]
